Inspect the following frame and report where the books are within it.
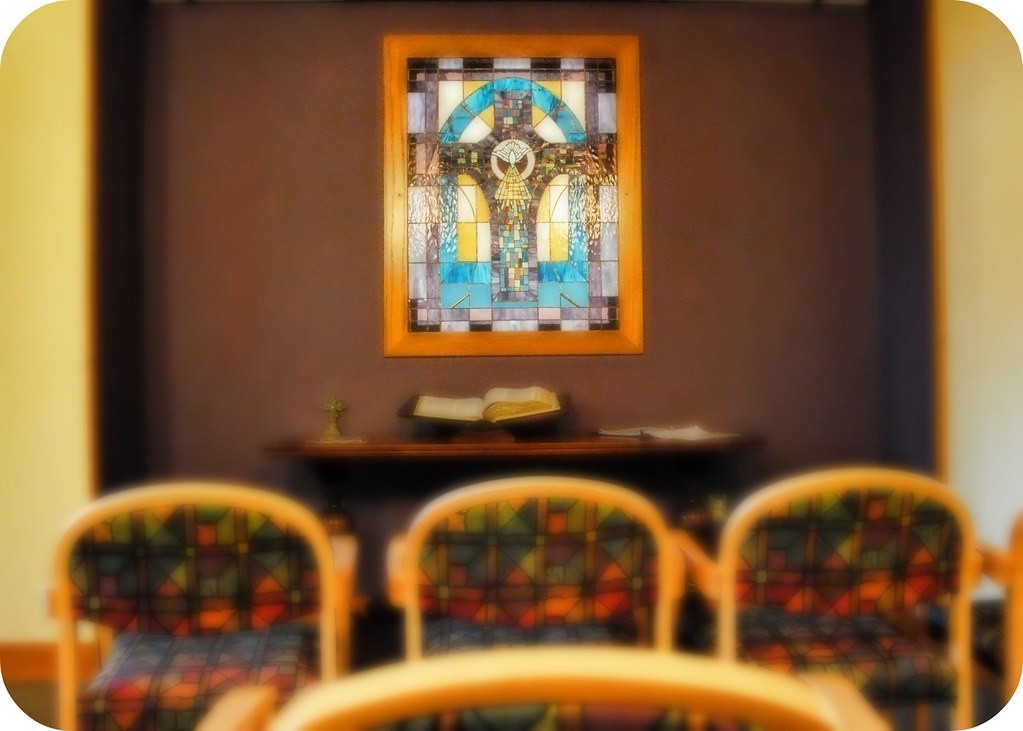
[397,386,570,430]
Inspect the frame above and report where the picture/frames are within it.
[384,34,645,356]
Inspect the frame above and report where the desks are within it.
[296,436,752,543]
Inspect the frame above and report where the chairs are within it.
[672,468,988,731]
[387,474,688,731]
[48,482,359,731]
